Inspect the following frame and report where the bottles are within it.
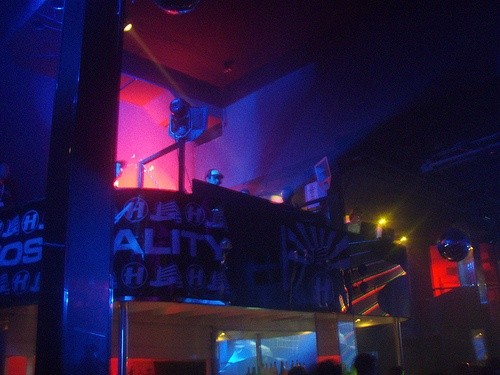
[247,360,306,375]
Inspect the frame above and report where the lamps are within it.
[168,97,194,139]
[206,169,224,186]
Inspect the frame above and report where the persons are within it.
[204,168,223,186]
[287,353,500,375]
[335,248,355,313]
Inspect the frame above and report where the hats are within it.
[205,169,224,179]
